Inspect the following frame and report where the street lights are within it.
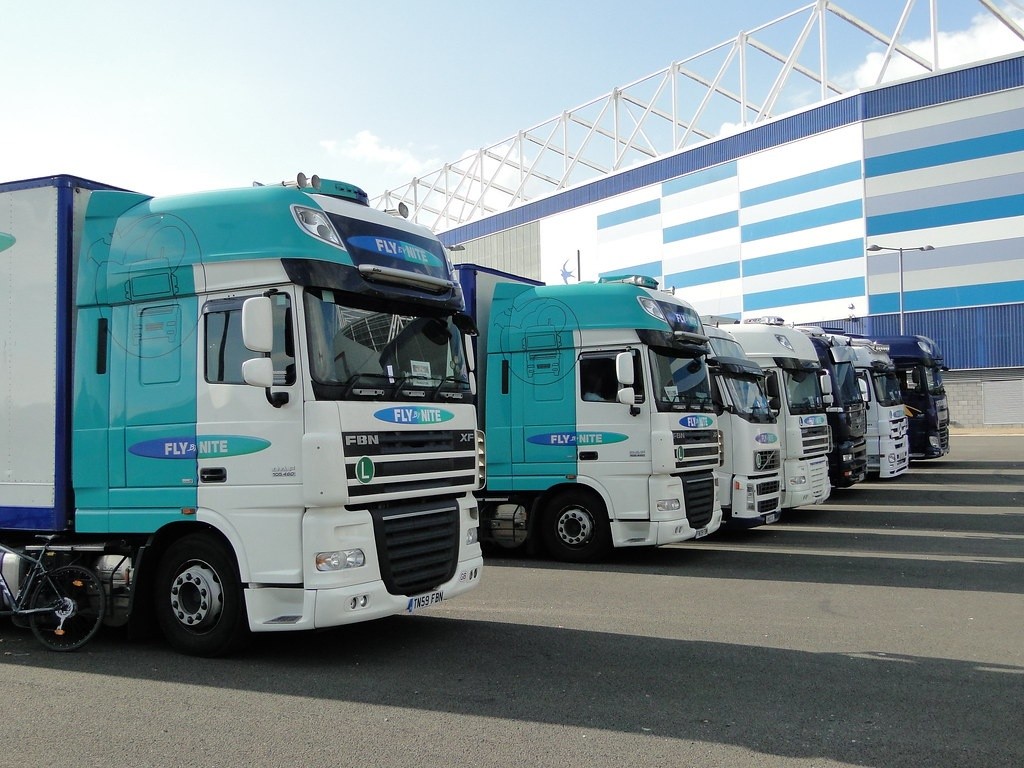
[865,244,935,336]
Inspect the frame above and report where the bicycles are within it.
[0,533,107,652]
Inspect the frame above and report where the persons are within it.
[583,369,613,402]
[269,311,331,374]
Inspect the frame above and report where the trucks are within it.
[449,262,726,565]
[691,322,782,531]
[711,316,832,512]
[864,334,952,460]
[791,327,870,492]
[1,170,483,656]
[844,334,910,478]
